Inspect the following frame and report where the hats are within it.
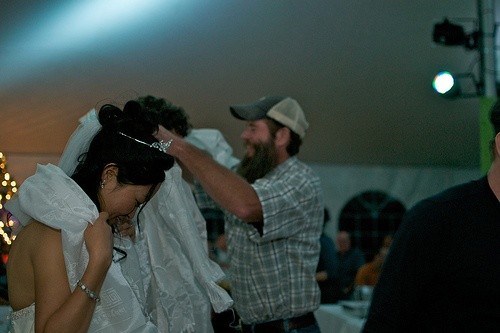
[230,96,309,138]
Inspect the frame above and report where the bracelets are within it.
[308,209,338,303]
[76,280,103,306]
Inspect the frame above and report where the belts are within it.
[240,311,316,333]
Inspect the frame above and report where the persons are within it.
[358,99,499,333]
[6,98,166,333]
[150,95,324,332]
[357,231,398,285]
[329,230,363,285]
